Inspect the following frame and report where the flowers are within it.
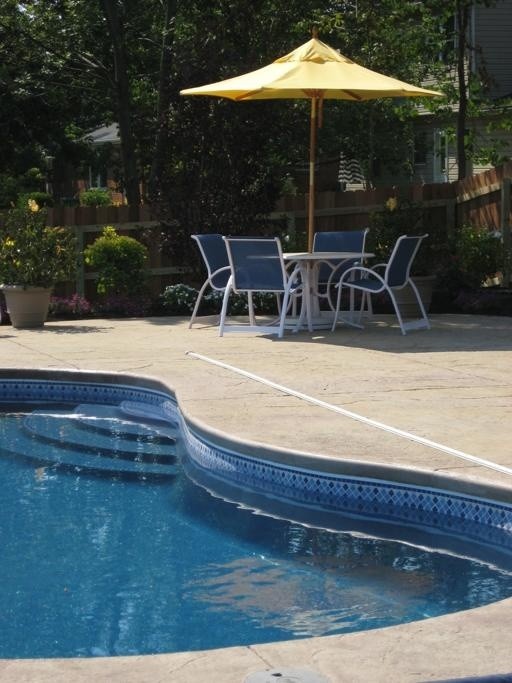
[0,193,77,287]
[369,197,429,275]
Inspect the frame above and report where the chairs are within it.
[189,227,432,338]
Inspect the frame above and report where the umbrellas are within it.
[176,19,448,250]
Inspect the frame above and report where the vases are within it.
[0,283,56,328]
